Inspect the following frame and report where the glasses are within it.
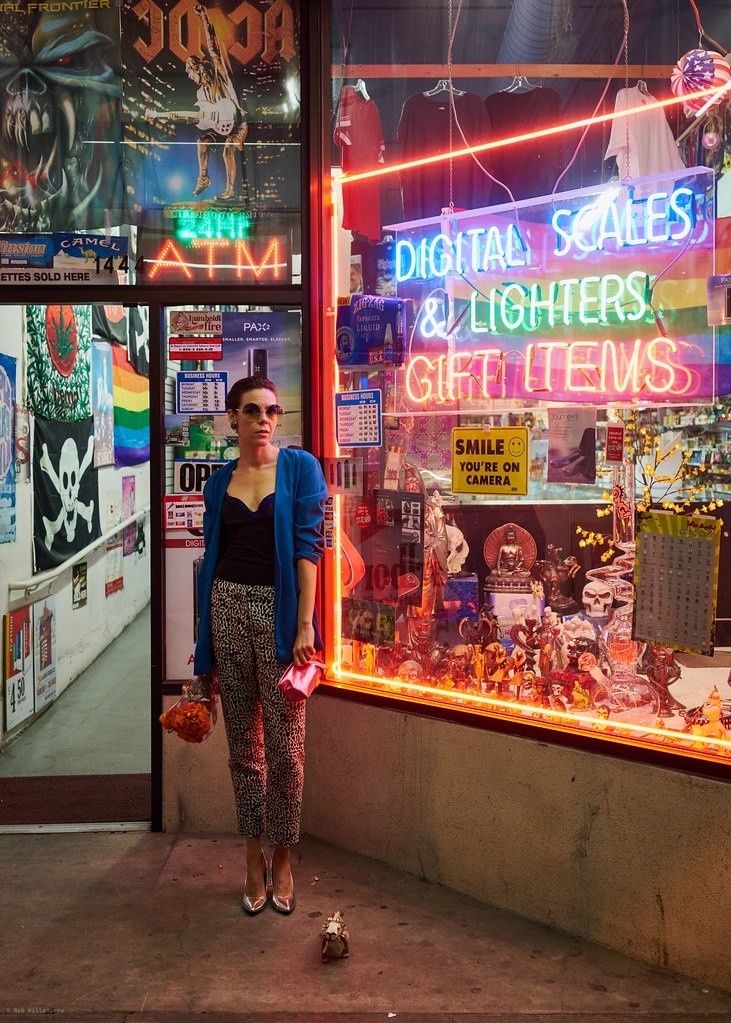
[232,402,285,420]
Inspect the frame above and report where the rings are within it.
[306,655,312,660]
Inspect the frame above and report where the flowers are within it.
[157,673,220,745]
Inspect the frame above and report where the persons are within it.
[692,692,727,737]
[539,540,570,603]
[349,265,363,293]
[192,376,327,917]
[169,4,248,202]
[362,603,610,720]
[337,333,352,358]
[490,526,530,578]
[646,650,687,711]
[398,461,448,654]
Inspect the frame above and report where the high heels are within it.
[270,859,296,913]
[243,848,269,915]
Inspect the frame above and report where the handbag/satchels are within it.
[276,655,329,702]
[159,673,218,744]
